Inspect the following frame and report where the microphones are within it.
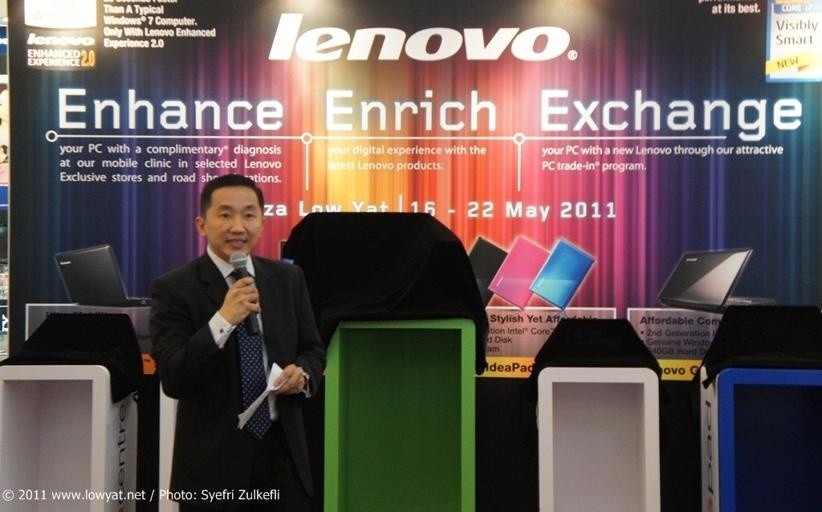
[229,250,261,337]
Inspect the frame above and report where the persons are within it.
[149,172,327,512]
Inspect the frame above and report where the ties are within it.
[228,270,271,439]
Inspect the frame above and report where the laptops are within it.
[657,248,776,315]
[54,245,152,307]
[465,235,595,310]
